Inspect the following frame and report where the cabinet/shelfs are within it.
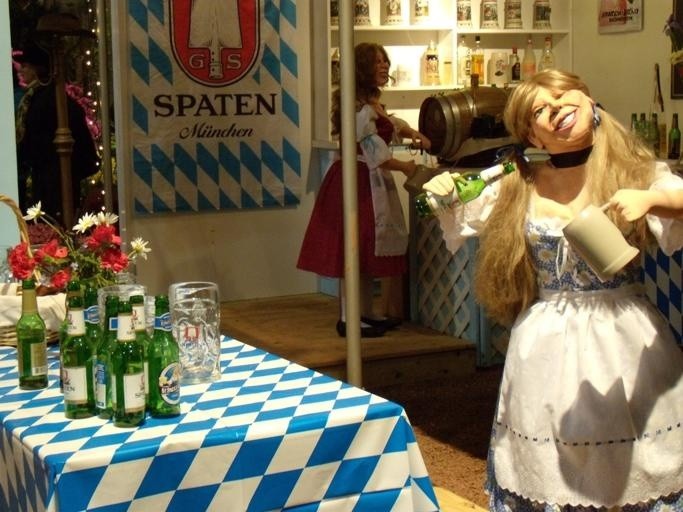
[408,186,512,369]
[311,1,573,150]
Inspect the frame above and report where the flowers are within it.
[8,201,152,294]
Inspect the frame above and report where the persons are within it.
[294,42,433,338]
[421,67,683,511]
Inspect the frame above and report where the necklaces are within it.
[549,146,593,169]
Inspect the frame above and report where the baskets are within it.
[0,194,68,347]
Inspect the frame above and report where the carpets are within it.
[434,488,494,512]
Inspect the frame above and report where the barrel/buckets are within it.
[418,86,515,165]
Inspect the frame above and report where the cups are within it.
[329,0,555,29]
[563,200,644,282]
[167,278,224,386]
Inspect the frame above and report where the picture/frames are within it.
[671,1,683,99]
[595,0,643,35]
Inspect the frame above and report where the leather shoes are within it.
[336,320,388,338]
[361,317,401,330]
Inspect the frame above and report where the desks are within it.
[0,332,440,512]
[220,293,504,508]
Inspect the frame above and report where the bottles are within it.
[666,112,679,160]
[146,292,181,422]
[420,33,555,87]
[411,161,517,220]
[628,108,660,158]
[58,278,146,430]
[15,278,48,392]
